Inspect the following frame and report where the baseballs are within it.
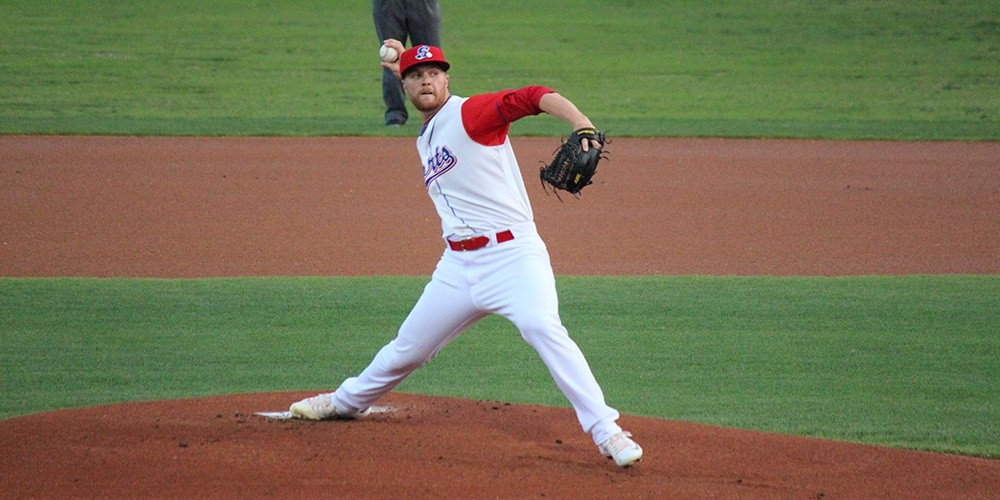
[379,44,400,63]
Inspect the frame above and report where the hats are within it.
[400,44,450,78]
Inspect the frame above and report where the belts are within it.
[448,230,514,251]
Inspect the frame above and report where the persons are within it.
[369,0,442,128]
[288,38,645,468]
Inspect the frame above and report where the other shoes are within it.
[385,111,407,128]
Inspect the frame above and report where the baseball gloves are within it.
[536,126,607,195]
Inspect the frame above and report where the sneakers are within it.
[599,431,643,468]
[290,394,355,420]
